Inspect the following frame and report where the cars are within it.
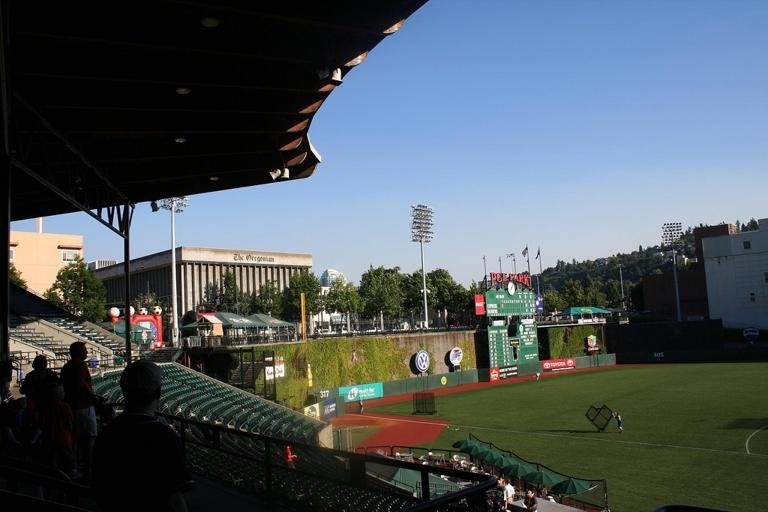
[313,326,381,333]
[272,328,300,339]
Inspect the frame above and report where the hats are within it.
[118,360,164,396]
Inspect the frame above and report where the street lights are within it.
[615,264,627,310]
[660,221,686,322]
[151,194,188,349]
[409,203,433,329]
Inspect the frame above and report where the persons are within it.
[503,479,515,509]
[90,360,190,511]
[19,355,60,453]
[524,489,538,512]
[313,326,322,337]
[284,443,298,471]
[612,411,624,433]
[60,341,108,464]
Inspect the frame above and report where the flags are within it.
[535,249,540,259]
[506,253,515,259]
[522,247,528,257]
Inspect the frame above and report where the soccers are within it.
[138,308,148,315]
[130,306,135,316]
[153,306,162,315]
[109,307,120,317]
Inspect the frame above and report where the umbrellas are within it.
[451,438,591,497]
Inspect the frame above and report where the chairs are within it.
[88,440,418,511]
[90,363,330,447]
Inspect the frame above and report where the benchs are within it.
[12,314,130,360]
